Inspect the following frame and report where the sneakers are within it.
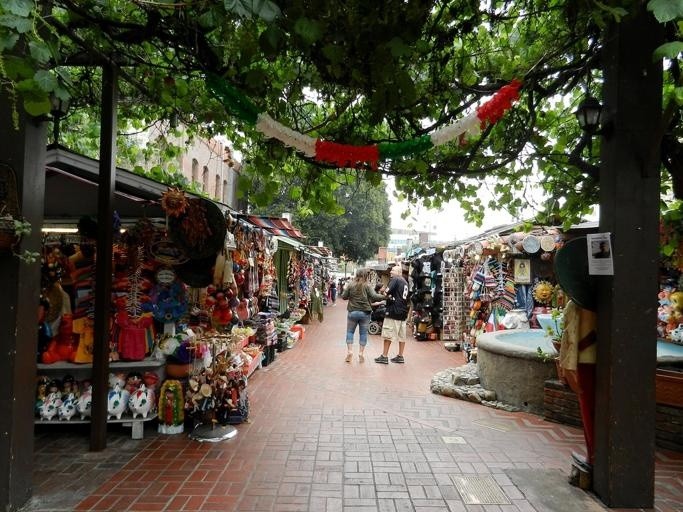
[391,355,404,363]
[374,355,388,364]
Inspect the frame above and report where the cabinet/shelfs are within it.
[33,354,166,440]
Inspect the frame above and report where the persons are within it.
[592,242,611,258]
[374,266,408,364]
[559,267,598,469]
[325,275,354,299]
[370,284,389,322]
[341,269,389,363]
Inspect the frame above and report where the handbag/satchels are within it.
[462,261,505,339]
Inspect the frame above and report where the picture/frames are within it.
[511,256,533,285]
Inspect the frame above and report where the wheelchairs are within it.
[368,306,386,335]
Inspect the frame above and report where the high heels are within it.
[357,352,365,363]
[345,351,354,362]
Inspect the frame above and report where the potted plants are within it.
[543,311,562,353]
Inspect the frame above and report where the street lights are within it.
[343,260,347,280]
